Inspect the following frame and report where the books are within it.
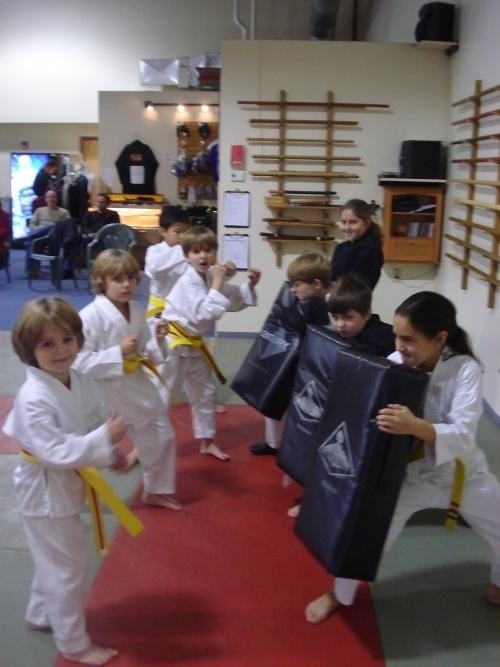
[406,221,435,238]
[265,196,291,208]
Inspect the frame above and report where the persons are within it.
[298,285,500,626]
[72,193,121,271]
[0,292,137,666]
[66,246,187,511]
[21,190,72,281]
[127,205,204,390]
[242,252,336,455]
[326,193,390,299]
[148,223,262,461]
[32,158,67,199]
[284,269,397,521]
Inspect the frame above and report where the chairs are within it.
[1,212,139,293]
